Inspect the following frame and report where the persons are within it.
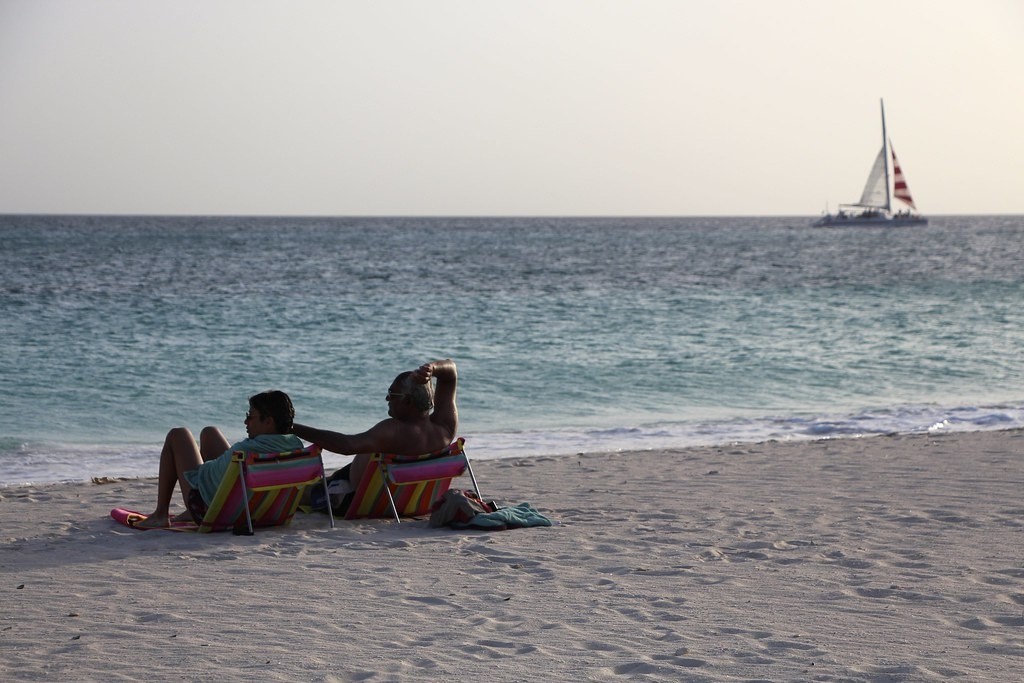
[134,390,304,527]
[292,359,458,501]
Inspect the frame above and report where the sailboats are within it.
[814,98,927,226]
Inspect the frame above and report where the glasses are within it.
[246,412,262,419]
[387,389,405,399]
